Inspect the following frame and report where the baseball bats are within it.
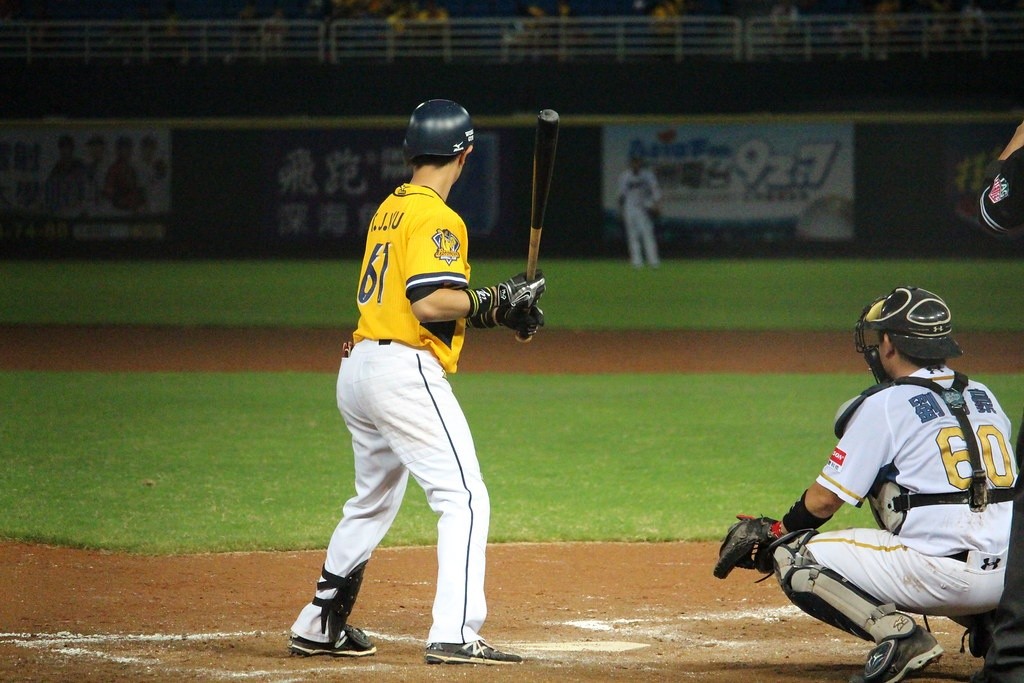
[513,109,561,344]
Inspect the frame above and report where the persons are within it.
[711,121,1024,683]
[0,0,992,94]
[289,101,546,671]
[616,146,659,271]
[0,131,173,233]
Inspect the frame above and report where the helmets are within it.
[406,100,474,159]
[866,286,963,360]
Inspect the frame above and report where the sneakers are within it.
[849,625,943,683]
[426,639,523,665]
[287,625,377,656]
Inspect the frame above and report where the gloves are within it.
[494,270,546,307]
[496,306,544,335]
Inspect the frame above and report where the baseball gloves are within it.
[712,516,782,580]
